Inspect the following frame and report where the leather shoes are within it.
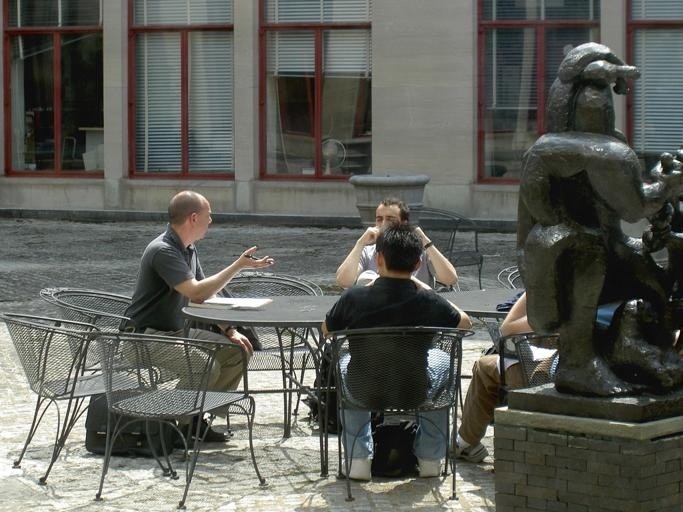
[169,430,194,449]
[192,419,224,441]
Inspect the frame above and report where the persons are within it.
[322,221,472,481]
[118,191,274,442]
[334,197,457,288]
[450,292,620,462]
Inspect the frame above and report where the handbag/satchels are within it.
[85,393,173,456]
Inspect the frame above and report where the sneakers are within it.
[449,440,490,462]
[340,458,372,480]
[322,416,341,433]
[416,456,444,478]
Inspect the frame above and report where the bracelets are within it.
[423,241,434,250]
[223,326,235,336]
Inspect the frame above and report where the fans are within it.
[319,139,347,175]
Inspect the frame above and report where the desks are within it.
[180,294,341,479]
[442,288,533,384]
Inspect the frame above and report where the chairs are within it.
[402,205,485,292]
[90,331,265,509]
[483,331,563,477]
[477,264,526,360]
[316,326,476,502]
[1,313,171,486]
[208,273,326,433]
[41,286,160,452]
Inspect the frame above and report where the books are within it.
[189,297,273,309]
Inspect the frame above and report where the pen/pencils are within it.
[231,307,264,311]
[244,254,272,264]
